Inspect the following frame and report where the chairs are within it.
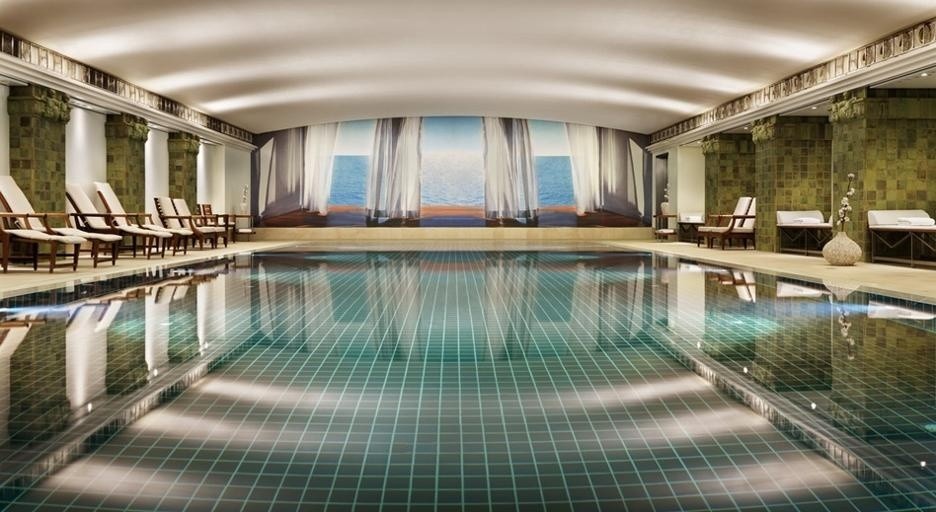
[653,195,757,250]
[0,175,256,272]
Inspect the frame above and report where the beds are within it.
[775,209,833,251]
[867,209,936,268]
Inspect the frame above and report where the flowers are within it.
[836,173,856,231]
[838,302,856,361]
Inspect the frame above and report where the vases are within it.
[822,232,862,266]
[822,267,864,302]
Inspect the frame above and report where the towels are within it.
[792,218,820,224]
[897,217,936,225]
